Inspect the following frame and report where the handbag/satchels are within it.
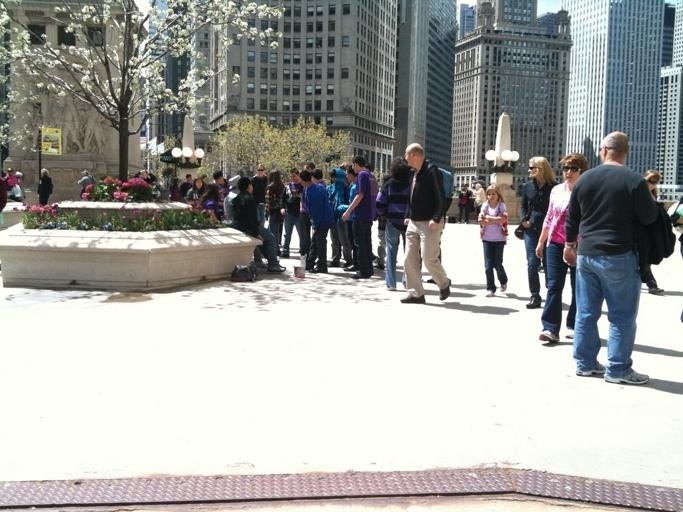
[231,265,257,281]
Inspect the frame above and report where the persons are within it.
[342,155,379,278]
[478,184,508,297]
[279,167,304,258]
[131,171,158,189]
[37,168,54,207]
[180,174,193,196]
[377,218,389,270]
[339,162,359,271]
[521,156,559,309]
[300,162,327,258]
[7,168,23,201]
[187,176,208,201]
[251,164,269,227]
[77,170,96,198]
[0,168,8,215]
[536,153,588,344]
[400,142,451,304]
[199,184,222,223]
[213,170,230,202]
[327,163,354,268]
[642,170,664,294]
[376,157,409,291]
[364,161,375,173]
[223,175,242,226]
[299,170,335,274]
[265,170,287,256]
[672,196,683,323]
[374,173,391,270]
[458,184,472,224]
[311,169,326,190]
[562,131,659,384]
[201,174,208,189]
[170,178,182,202]
[473,183,486,214]
[227,177,286,274]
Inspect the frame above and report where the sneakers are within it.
[501,282,507,292]
[269,264,286,273]
[603,368,650,384]
[539,330,560,343]
[651,286,664,295]
[485,290,496,297]
[388,284,398,291]
[565,329,575,338]
[577,361,606,377]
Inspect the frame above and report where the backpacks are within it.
[467,197,475,212]
[440,168,453,214]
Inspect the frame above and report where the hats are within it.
[228,175,241,189]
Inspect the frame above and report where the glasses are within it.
[563,166,582,173]
[257,169,266,171]
[648,180,660,186]
[529,167,538,170]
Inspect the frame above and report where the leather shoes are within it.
[304,258,385,279]
[439,279,451,299]
[526,297,541,308]
[401,295,425,304]
[428,279,436,284]
